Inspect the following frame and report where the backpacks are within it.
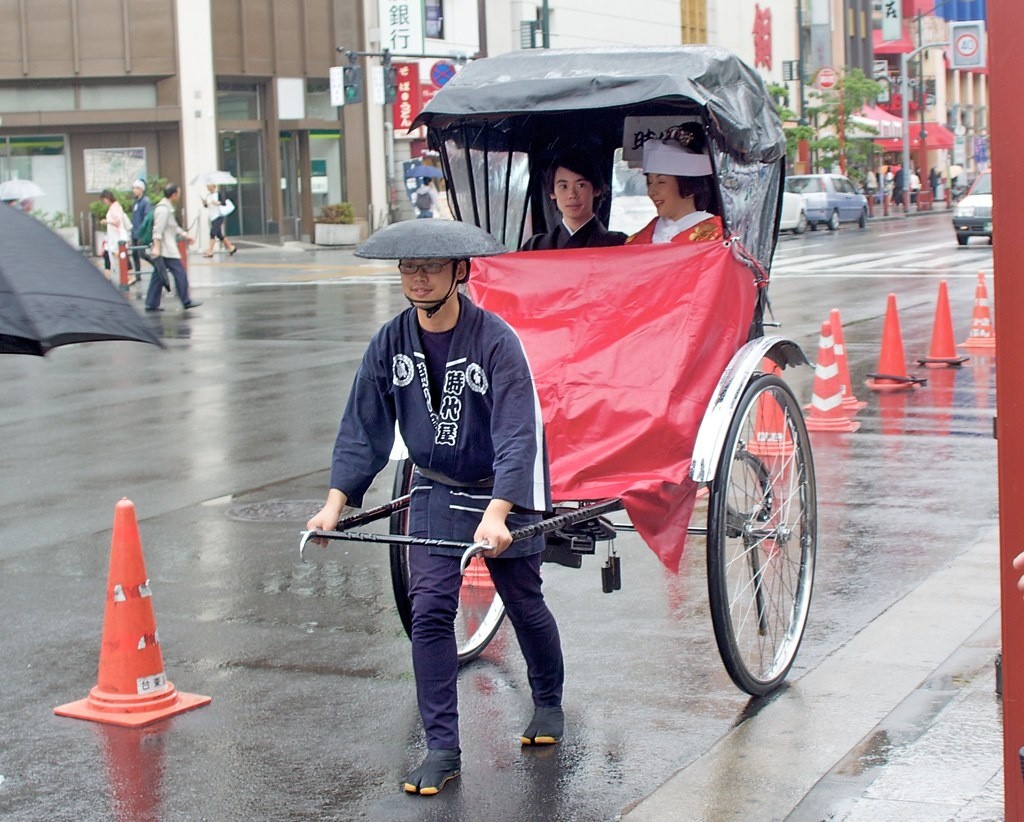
[137,207,154,249]
[416,188,432,210]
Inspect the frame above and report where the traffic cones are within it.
[803,307,868,412]
[916,279,971,370]
[747,357,793,455]
[804,320,862,434]
[461,555,496,591]
[956,271,998,349]
[51,495,213,730]
[863,293,922,394]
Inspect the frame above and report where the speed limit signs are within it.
[955,33,980,58]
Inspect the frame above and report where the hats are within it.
[353,219,509,260]
[642,138,713,177]
[132,180,145,191]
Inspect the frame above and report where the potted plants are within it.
[89,174,180,256]
[314,202,359,246]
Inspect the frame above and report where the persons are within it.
[516,147,628,252]
[131,179,152,285]
[622,121,728,245]
[145,184,202,312]
[305,217,564,797]
[3,197,33,214]
[100,190,136,285]
[863,163,942,205]
[201,184,237,257]
[415,176,437,218]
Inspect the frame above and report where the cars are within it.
[784,174,869,230]
[953,168,992,246]
[779,176,808,235]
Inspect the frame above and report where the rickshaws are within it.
[298,44,819,696]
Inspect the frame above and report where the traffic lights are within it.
[383,67,397,104]
[343,66,363,105]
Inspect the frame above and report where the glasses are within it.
[397,258,456,274]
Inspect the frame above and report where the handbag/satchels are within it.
[102,250,132,270]
[220,199,235,217]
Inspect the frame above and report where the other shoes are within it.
[145,304,166,311]
[229,247,237,255]
[203,253,213,257]
[521,706,565,746]
[185,302,202,310]
[403,747,462,795]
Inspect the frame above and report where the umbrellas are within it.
[404,165,446,178]
[0,180,45,200]
[0,204,166,357]
[149,242,171,293]
[188,169,240,186]
